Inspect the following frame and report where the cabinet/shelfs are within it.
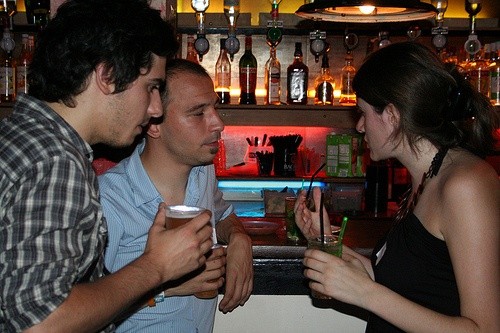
[159,17,500,182]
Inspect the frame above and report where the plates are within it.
[240,219,279,236]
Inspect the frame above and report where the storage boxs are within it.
[326,133,367,178]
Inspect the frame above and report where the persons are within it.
[96,58,253,333]
[294,38,500,333]
[0,0,214,333]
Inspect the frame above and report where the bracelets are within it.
[154,291,164,303]
[149,299,155,307]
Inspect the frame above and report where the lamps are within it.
[295,0,439,23]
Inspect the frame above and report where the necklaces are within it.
[395,143,449,224]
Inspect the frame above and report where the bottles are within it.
[338,52,360,107]
[344,0,500,110]
[363,153,409,220]
[0,0,52,101]
[238,36,258,105]
[191,0,282,59]
[314,53,335,106]
[309,23,327,62]
[264,46,282,106]
[212,37,233,105]
[185,36,201,64]
[286,41,309,105]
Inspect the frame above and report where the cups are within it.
[272,146,297,178]
[306,234,342,299]
[164,204,206,232]
[257,158,272,177]
[194,246,221,300]
[247,146,273,163]
[284,197,309,245]
